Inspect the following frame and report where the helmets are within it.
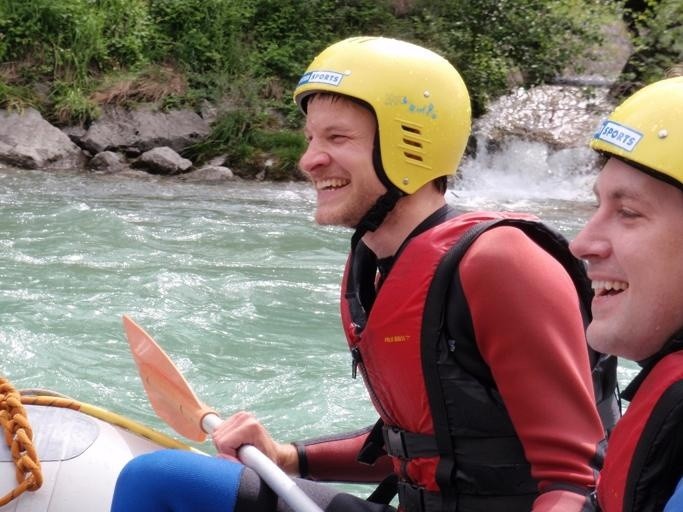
[588,74,683,189]
[291,35,474,197]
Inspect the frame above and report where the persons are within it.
[569,75,683,512]
[110,34,622,512]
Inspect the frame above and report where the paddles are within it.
[123,314,323,512]
